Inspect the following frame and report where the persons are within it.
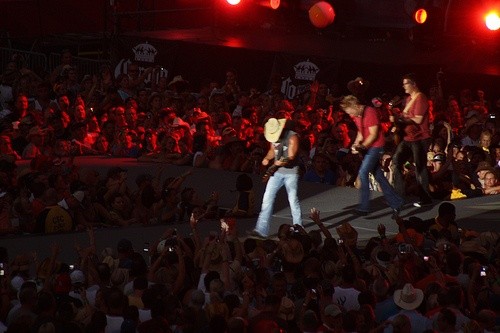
[0,47,500,333]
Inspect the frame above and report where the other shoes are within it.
[246,228,267,240]
[414,200,433,207]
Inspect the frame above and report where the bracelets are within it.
[380,236,387,239]
[320,223,323,227]
[302,302,306,307]
[352,255,356,258]
[191,226,197,231]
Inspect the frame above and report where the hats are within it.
[278,239,304,263]
[264,117,288,143]
[394,284,424,309]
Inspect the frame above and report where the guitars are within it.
[336,147,364,167]
[255,159,289,184]
[385,96,404,136]
